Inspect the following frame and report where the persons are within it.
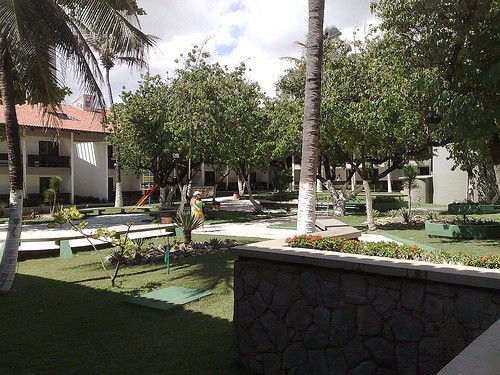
[193,193,204,229]
[190,196,195,218]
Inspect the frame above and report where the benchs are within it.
[185,183,218,204]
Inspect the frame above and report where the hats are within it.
[193,190,202,196]
